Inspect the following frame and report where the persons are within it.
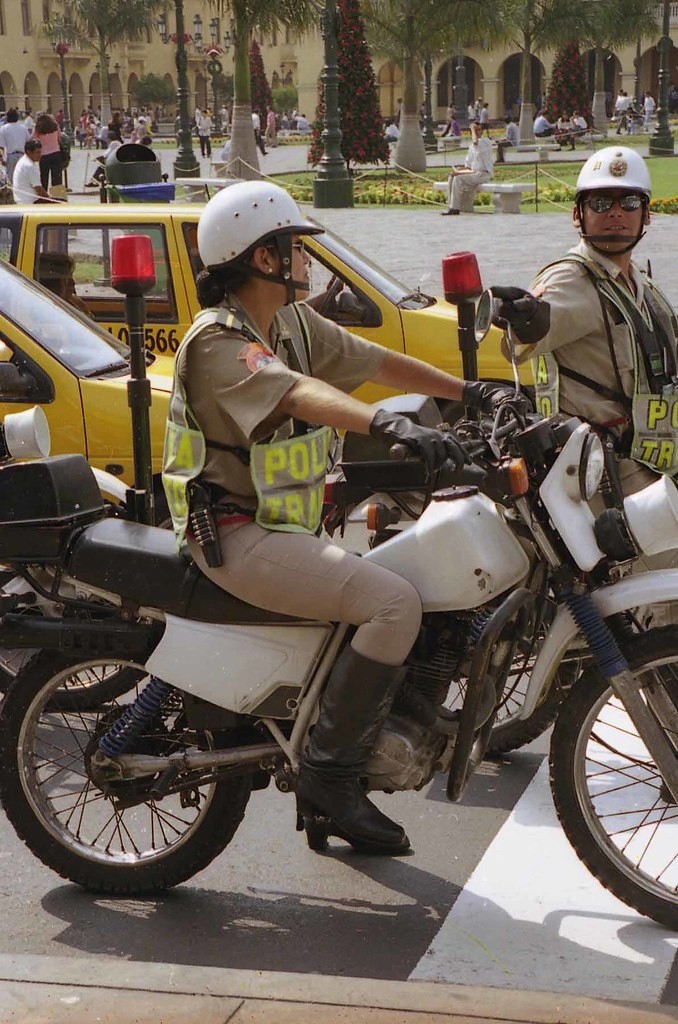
[467,97,492,140]
[264,105,277,147]
[494,116,519,163]
[488,146,678,801]
[440,122,493,215]
[382,98,427,151]
[160,181,531,858]
[283,110,308,130]
[194,106,214,134]
[614,90,656,135]
[84,131,123,187]
[441,102,461,136]
[252,108,268,155]
[196,110,212,158]
[0,106,72,204]
[78,105,160,149]
[533,110,588,151]
[38,253,94,318]
[139,136,169,182]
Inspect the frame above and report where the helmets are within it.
[196,180,324,270]
[574,145,652,203]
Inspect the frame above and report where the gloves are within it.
[487,286,551,343]
[369,408,472,479]
[463,380,534,426]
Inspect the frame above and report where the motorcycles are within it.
[0,232,678,934]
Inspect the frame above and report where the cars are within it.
[0,257,178,513]
[0,204,537,429]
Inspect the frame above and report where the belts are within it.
[12,151,24,155]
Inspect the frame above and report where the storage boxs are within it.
[1,454,104,568]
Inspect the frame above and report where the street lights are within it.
[47,31,75,147]
[192,29,232,132]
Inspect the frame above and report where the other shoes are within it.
[442,208,460,215]
[495,159,505,163]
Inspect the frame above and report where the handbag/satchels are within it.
[195,127,199,134]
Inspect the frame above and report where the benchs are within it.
[437,135,564,163]
[435,180,537,217]
[176,177,246,204]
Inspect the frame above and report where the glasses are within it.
[266,237,305,254]
[582,191,646,213]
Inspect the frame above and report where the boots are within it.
[297,642,408,847]
